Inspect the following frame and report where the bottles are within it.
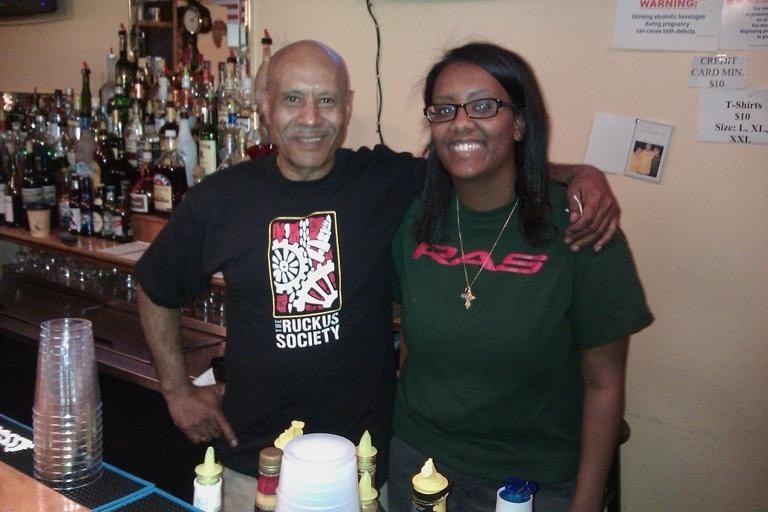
[354,445,377,488]
[194,464,224,511]
[410,472,451,512]
[361,488,378,511]
[148,8,159,21]
[1,23,274,244]
[254,447,282,512]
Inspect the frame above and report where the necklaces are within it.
[456,185,520,314]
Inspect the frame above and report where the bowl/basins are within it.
[277,433,361,511]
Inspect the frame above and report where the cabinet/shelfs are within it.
[130,0,179,75]
[0,213,229,398]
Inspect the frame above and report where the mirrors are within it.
[125,1,251,90]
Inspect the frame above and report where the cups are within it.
[14,246,226,324]
[33,319,104,489]
[495,486,533,511]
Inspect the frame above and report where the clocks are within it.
[181,6,203,37]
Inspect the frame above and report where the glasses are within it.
[424,98,516,123]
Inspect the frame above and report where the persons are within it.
[628,142,660,179]
[129,38,622,512]
[385,40,655,512]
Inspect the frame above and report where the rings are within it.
[199,434,209,442]
